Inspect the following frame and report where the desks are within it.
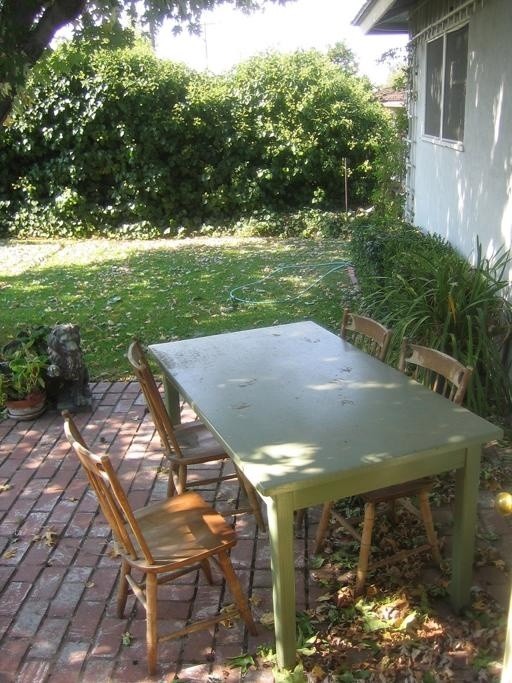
[148,321,503,670]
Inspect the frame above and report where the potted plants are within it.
[0,326,49,420]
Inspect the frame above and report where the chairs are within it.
[128,339,266,534]
[310,335,474,596]
[61,408,259,676]
[290,308,394,531]
